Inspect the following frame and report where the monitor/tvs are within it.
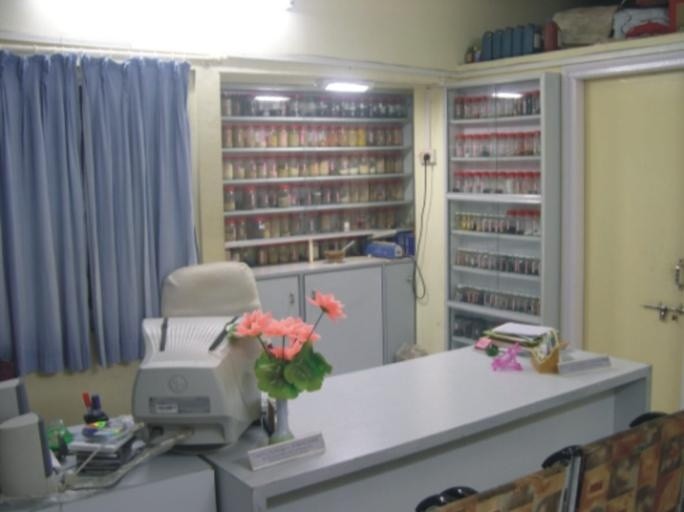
[130,316,263,447]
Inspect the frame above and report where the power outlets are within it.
[420,148,435,164]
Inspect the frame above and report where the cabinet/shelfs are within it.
[449,70,561,353]
[241,269,381,378]
[221,60,413,267]
[380,264,416,361]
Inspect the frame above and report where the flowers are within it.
[223,286,347,402]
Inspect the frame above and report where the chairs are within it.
[567,407,684,512]
[427,451,572,511]
[159,259,259,313]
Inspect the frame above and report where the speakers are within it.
[0,411,56,499]
[0,378,29,421]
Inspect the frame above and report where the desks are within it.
[0,414,221,512]
[197,336,652,512]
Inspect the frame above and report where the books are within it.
[472,322,557,357]
[69,418,145,476]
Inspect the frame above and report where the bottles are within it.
[44,407,72,451]
[447,89,541,340]
[79,391,108,428]
[220,88,405,267]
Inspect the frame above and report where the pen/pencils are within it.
[82,391,101,416]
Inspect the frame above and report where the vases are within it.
[266,392,295,444]
